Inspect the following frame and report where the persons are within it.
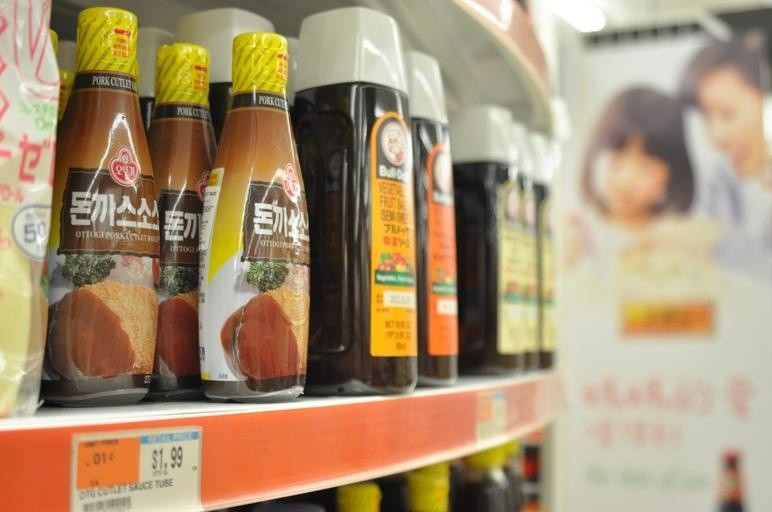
[552,84,696,264]
[681,38,771,276]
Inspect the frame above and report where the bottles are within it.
[40,7,554,512]
[710,452,748,512]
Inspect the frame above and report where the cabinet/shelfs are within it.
[0,0,554,512]
